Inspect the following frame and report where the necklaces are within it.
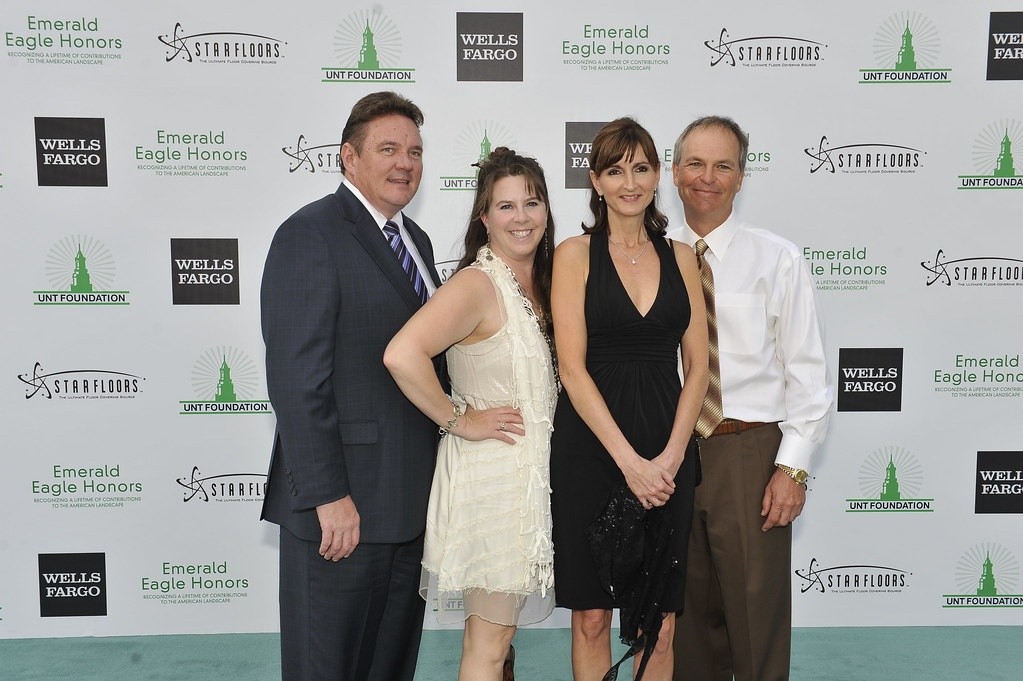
[610,231,648,264]
[505,266,563,396]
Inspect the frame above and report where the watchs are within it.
[778,463,809,485]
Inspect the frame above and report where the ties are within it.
[383,221,431,305]
[693,239,722,439]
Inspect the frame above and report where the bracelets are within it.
[439,397,460,435]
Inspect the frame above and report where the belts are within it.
[692,422,775,438]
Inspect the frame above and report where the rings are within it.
[499,420,507,431]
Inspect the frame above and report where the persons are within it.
[383,148,563,680]
[260,88,453,681]
[661,115,833,681]
[550,118,710,681]
